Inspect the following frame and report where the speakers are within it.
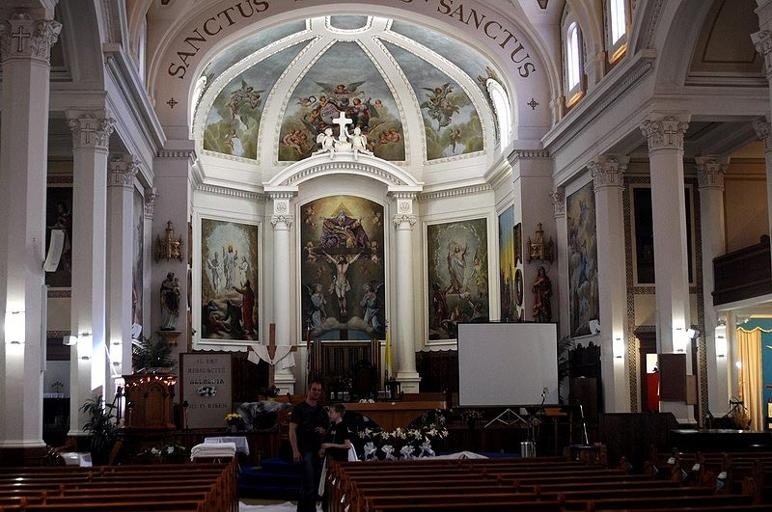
[659,355,686,401]
[573,377,597,442]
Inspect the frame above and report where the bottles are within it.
[703,415,711,431]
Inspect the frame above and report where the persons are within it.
[46,201,70,272]
[532,266,554,322]
[447,126,463,153]
[225,127,236,150]
[223,85,253,107]
[160,272,181,330]
[435,88,452,116]
[429,94,459,119]
[205,245,256,336]
[230,93,261,112]
[281,82,402,159]
[433,242,484,336]
[308,282,325,329]
[289,380,329,512]
[317,210,368,249]
[314,404,352,512]
[321,249,362,317]
[360,284,383,333]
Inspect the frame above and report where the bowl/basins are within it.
[544,407,561,414]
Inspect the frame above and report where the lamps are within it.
[60,334,79,348]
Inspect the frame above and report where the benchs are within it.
[0,457,248,511]
